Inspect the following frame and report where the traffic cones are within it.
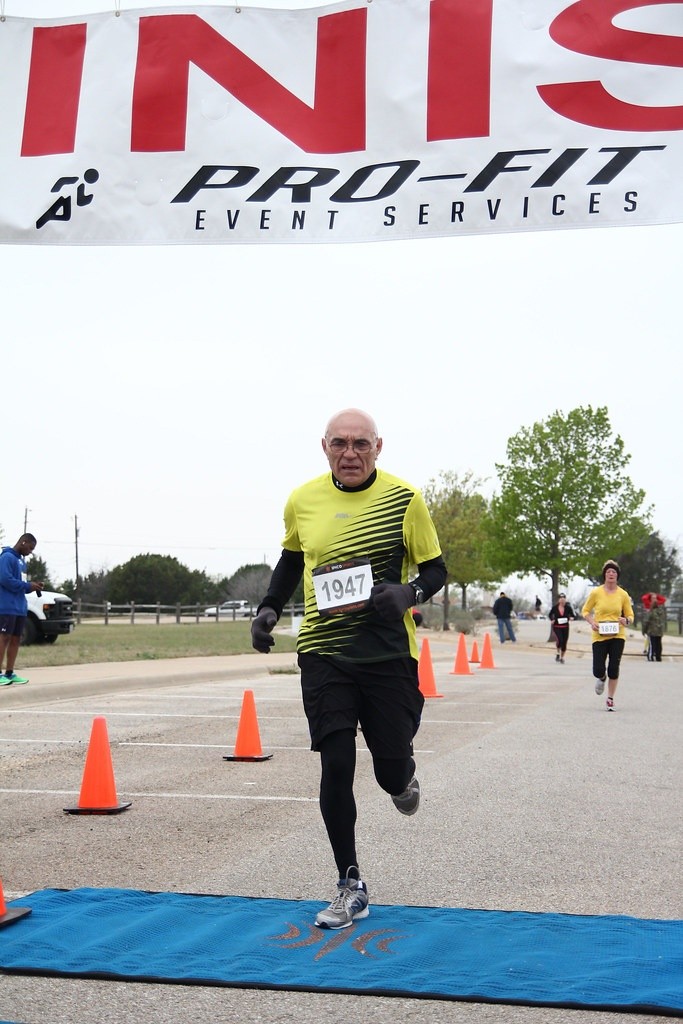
[476,633,498,669]
[468,640,482,663]
[222,689,277,761]
[62,715,138,817]
[0,876,31,929]
[449,632,474,675]
[416,638,444,697]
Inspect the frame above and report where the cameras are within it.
[35,583,43,597]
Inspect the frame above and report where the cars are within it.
[510,611,548,621]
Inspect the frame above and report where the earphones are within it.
[22,542,26,544]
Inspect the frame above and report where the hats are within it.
[601,559,621,581]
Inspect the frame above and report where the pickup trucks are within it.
[204,600,259,617]
[17,590,76,646]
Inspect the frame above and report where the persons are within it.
[581,560,634,711]
[642,593,666,653]
[251,407,448,929]
[643,602,667,661]
[412,609,423,626]
[535,595,541,619]
[548,593,576,663]
[0,533,44,685]
[493,592,516,643]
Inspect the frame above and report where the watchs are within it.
[408,582,424,605]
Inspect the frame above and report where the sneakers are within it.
[595,679,605,695]
[556,654,560,661]
[4,673,30,685]
[315,865,369,929]
[0,673,11,685]
[561,658,565,664]
[606,697,616,712]
[392,774,420,816]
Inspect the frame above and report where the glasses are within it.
[559,596,567,599]
[325,437,377,454]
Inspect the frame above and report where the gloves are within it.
[372,584,416,624]
[251,607,277,655]
